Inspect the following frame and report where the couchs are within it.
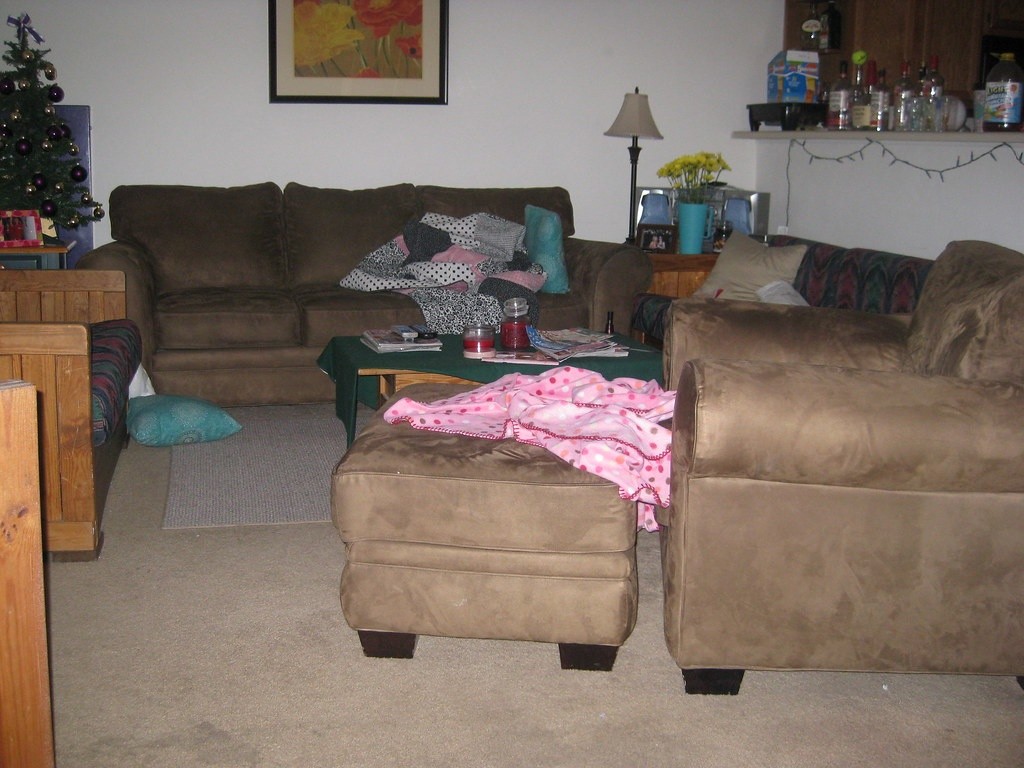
[72,181,656,403]
[664,239,1024,697]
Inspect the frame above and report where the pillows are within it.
[127,395,242,446]
[695,230,808,305]
[524,204,571,294]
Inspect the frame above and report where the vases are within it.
[677,202,713,254]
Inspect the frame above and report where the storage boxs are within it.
[767,49,820,102]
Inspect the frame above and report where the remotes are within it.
[390,324,418,339]
[409,325,437,339]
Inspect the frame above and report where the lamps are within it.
[604,87,664,244]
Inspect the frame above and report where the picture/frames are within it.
[268,0,450,104]
[0,209,44,248]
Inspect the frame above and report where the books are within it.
[360,328,443,354]
[481,325,630,366]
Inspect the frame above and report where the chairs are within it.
[0,268,143,561]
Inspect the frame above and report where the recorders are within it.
[634,182,770,238]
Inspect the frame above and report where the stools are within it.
[329,383,639,669]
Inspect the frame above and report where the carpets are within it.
[161,402,378,530]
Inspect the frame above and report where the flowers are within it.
[655,152,732,203]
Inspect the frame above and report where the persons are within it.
[649,235,665,249]
[0,217,24,241]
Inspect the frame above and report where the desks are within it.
[0,239,76,269]
[643,254,716,300]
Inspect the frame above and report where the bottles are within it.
[605,311,615,333]
[801,0,843,50]
[820,51,1024,133]
[711,190,752,251]
[463,297,531,360]
